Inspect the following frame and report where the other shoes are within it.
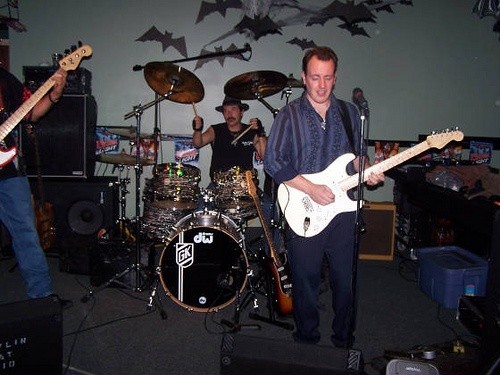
[48,293,73,309]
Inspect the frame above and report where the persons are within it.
[192,96,282,257]
[0,66,73,309]
[264,47,385,350]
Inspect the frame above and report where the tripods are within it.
[81,62,161,302]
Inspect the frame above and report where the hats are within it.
[215,96,249,112]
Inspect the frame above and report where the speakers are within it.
[221,334,364,374]
[19,95,98,180]
[38,178,120,258]
[1,298,68,375]
[355,205,397,262]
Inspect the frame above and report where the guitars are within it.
[278,127,464,237]
[0,39,94,168]
[245,170,293,321]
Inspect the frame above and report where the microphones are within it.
[352,88,369,111]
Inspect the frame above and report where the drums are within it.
[212,168,263,222]
[142,193,199,243]
[152,163,201,200]
[158,210,250,313]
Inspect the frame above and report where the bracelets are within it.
[48,91,59,104]
[256,132,266,137]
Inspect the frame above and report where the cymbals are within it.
[93,153,157,166]
[223,70,288,100]
[286,77,305,89]
[105,128,151,139]
[144,61,205,104]
